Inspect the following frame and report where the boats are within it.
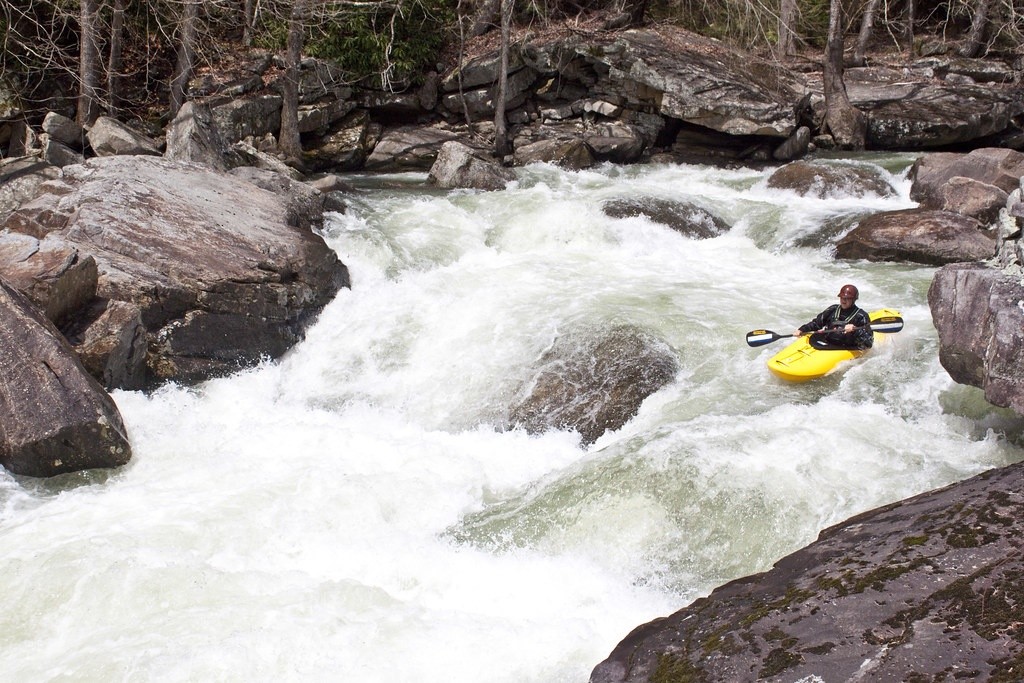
[766,308,902,384]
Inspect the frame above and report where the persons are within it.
[793,285,874,348]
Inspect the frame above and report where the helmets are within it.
[838,285,859,299]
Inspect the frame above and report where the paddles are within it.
[746,316,904,348]
